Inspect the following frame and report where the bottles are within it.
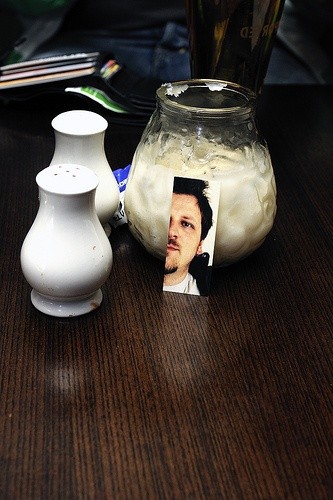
[38,109,121,240]
[20,161,114,317]
[124,78,277,266]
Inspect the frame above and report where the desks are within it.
[0,82,333,500]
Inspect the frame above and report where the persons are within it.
[162,177,215,297]
[1,0,326,86]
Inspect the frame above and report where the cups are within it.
[184,0,285,107]
